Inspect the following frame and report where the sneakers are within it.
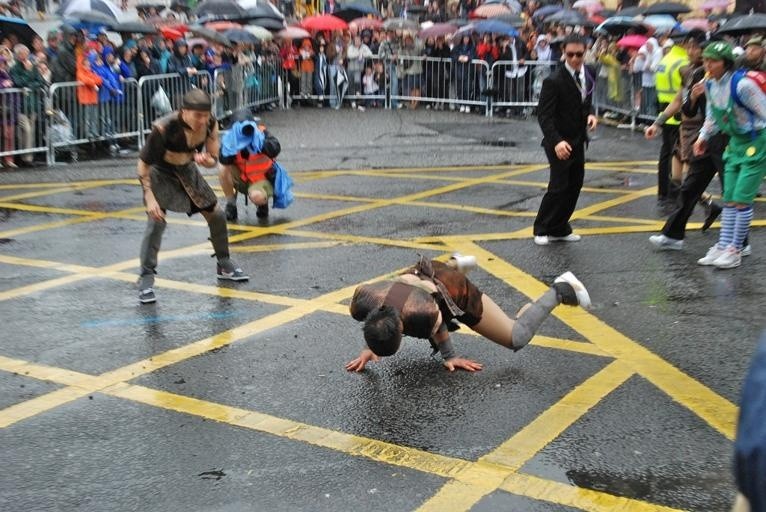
[225,203,237,221]
[217,265,250,281]
[698,238,751,268]
[555,271,592,309]
[256,205,268,217]
[535,234,580,246]
[452,255,475,273]
[648,234,685,250]
[700,203,722,231]
[138,288,156,302]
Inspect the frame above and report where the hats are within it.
[703,41,734,62]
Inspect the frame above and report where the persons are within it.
[133,89,250,305]
[644,28,723,233]
[727,327,766,512]
[0,0,765,170]
[344,252,594,374]
[220,117,280,221]
[530,34,598,247]
[694,41,766,268]
[648,41,752,258]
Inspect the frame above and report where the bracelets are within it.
[656,110,669,127]
[138,173,154,192]
[436,336,455,360]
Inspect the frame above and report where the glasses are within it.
[566,52,584,58]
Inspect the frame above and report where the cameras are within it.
[242,125,253,136]
